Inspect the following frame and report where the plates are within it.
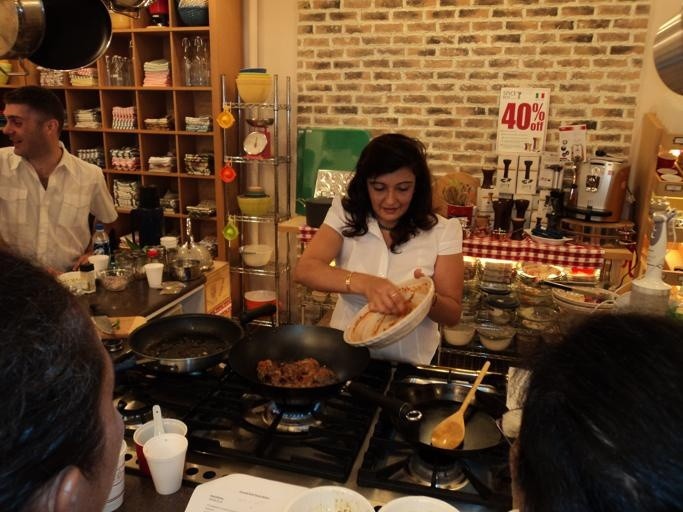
[342,277,435,350]
[524,228,573,246]
[552,287,619,312]
[288,486,459,512]
[517,264,568,278]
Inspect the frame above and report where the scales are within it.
[242,117,274,160]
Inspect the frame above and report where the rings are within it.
[390,291,401,299]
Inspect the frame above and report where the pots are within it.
[229,322,423,423]
[401,383,502,455]
[126,302,277,373]
[0,0,149,71]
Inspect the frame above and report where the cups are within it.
[101,418,188,512]
[88,255,109,279]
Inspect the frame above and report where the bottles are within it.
[105,41,134,86]
[93,223,111,259]
[181,35,209,86]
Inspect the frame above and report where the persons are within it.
[296,134,464,365]
[1,84,121,272]
[0,229,124,512]
[510,302,683,512]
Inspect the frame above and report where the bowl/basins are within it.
[443,282,558,351]
[235,67,277,310]
[58,260,202,296]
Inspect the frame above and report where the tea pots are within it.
[175,218,214,271]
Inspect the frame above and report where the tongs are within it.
[541,280,600,296]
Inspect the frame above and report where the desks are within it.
[37,251,229,336]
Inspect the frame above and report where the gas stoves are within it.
[104,313,515,502]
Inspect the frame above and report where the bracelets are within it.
[345,271,357,295]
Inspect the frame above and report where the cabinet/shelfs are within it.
[0,2,217,253]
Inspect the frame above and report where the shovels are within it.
[431,360,491,450]
